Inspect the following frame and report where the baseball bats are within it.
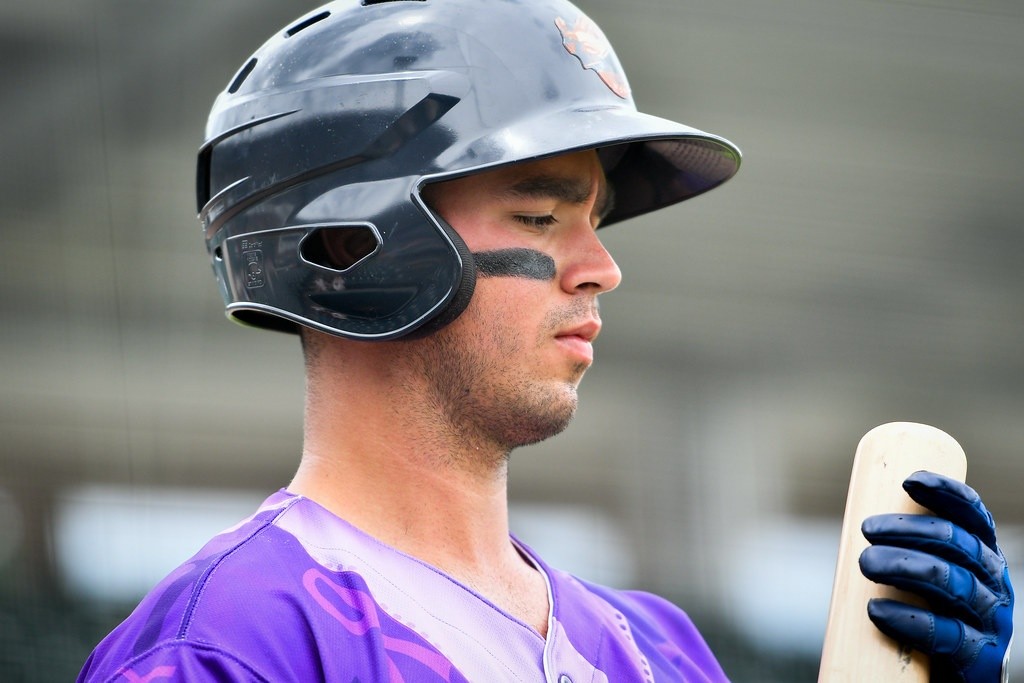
[816,421,969,683]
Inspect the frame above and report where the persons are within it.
[71,0,1016,683]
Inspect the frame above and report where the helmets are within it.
[196,0,743,343]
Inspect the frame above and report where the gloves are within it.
[857,470,1015,683]
